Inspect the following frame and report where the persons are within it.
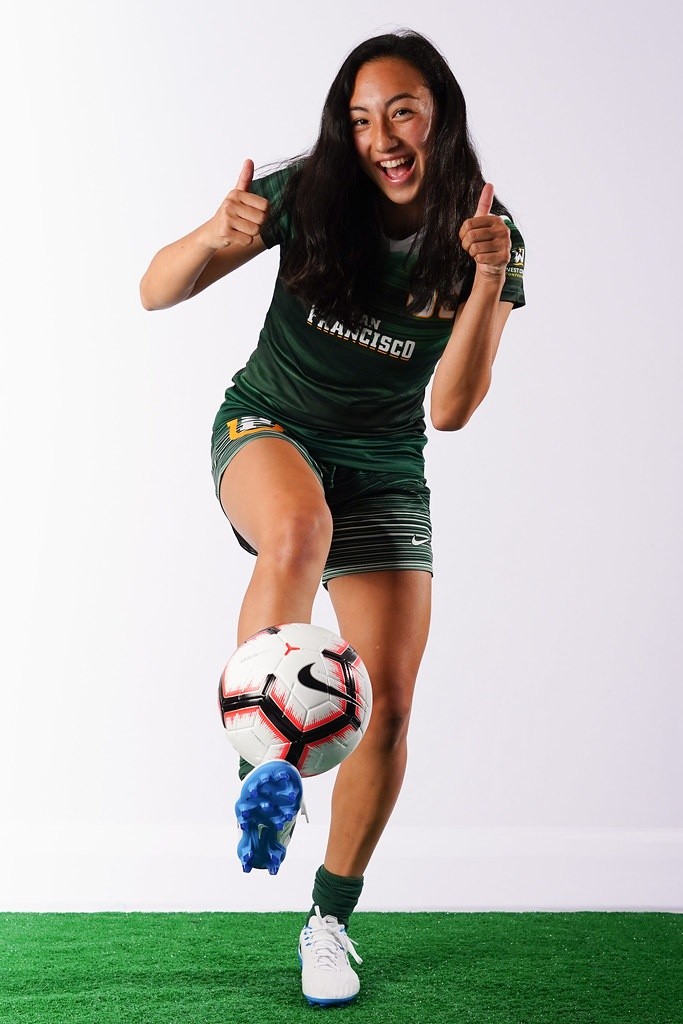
[139,32,526,1008]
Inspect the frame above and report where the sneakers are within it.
[235,758,310,877]
[297,905,364,1005]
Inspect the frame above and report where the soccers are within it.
[217,622,373,777]
